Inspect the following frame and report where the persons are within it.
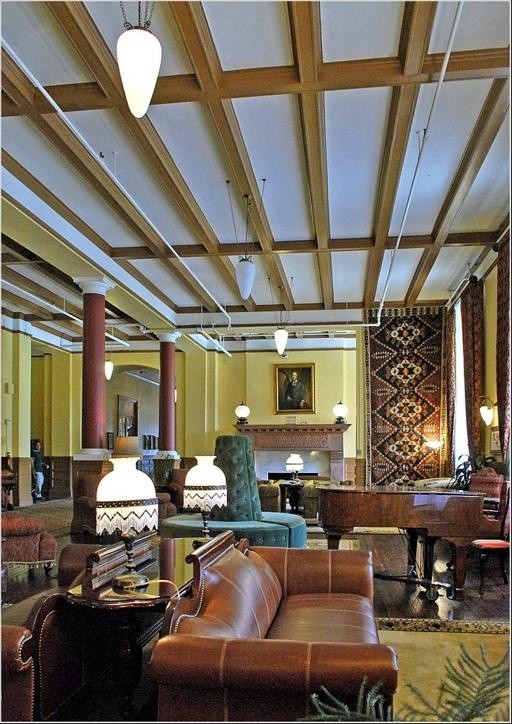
[286,370,306,409]
[31,441,49,500]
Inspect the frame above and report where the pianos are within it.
[315,483,500,599]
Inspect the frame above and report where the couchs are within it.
[2,542,113,721]
[167,468,200,513]
[70,473,178,544]
[149,529,399,721]
[1,508,58,594]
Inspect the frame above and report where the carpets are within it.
[374,617,510,721]
[303,537,361,551]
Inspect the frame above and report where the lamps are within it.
[94,455,160,593]
[478,393,498,427]
[181,455,229,539]
[226,178,265,301]
[286,453,305,484]
[267,276,294,356]
[331,399,347,424]
[104,328,114,381]
[234,400,251,425]
[116,1,163,120]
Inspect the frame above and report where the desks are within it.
[67,538,216,721]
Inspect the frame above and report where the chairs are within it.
[157,435,307,595]
[257,480,353,527]
[445,467,511,595]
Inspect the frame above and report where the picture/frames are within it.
[107,432,115,450]
[272,361,317,415]
[490,427,502,455]
